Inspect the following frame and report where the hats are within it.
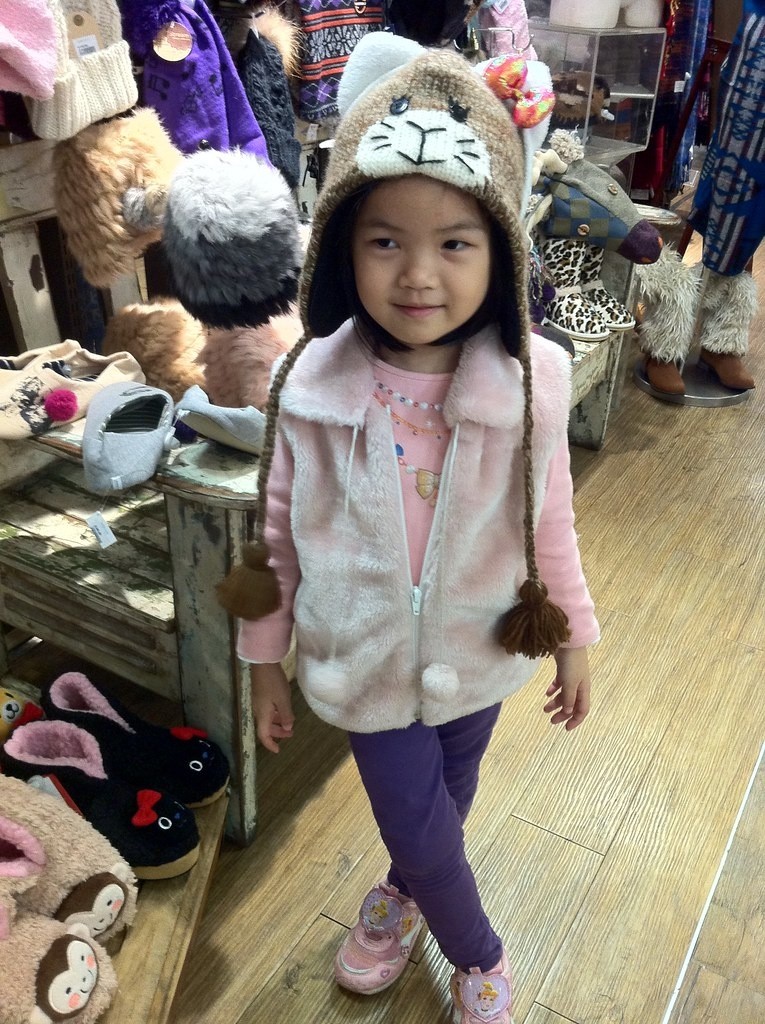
[219,32,574,659]
[1,0,303,326]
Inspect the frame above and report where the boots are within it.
[585,242,636,331]
[699,270,756,392]
[634,245,694,395]
[538,238,611,342]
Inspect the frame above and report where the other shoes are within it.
[0,337,147,440]
[176,388,267,454]
[83,381,175,494]
[0,664,231,1024]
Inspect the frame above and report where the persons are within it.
[214,33,599,1024]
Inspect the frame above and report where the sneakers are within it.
[336,881,426,995]
[450,947,513,1024]
